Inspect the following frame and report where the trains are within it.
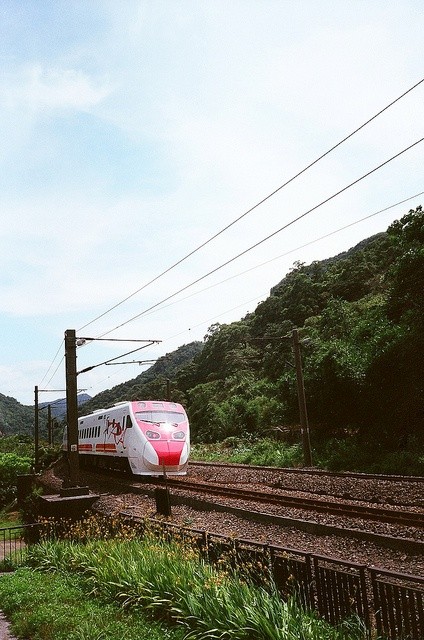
[62,400,190,479]
